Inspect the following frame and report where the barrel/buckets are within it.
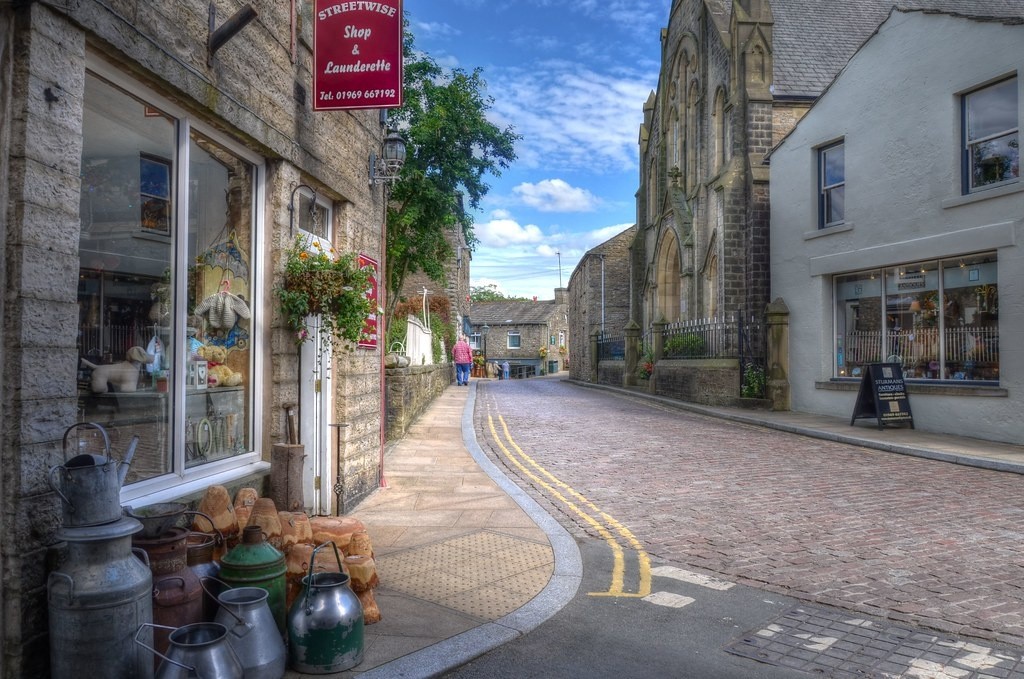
[287,540,367,675]
[220,524,287,646]
[186,533,221,620]
[199,575,286,679]
[133,526,203,672]
[135,623,246,679]
[47,534,154,679]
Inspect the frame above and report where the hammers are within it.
[282,401,297,444]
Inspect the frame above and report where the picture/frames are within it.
[140,151,173,204]
[138,195,172,236]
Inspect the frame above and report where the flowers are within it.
[279,234,380,348]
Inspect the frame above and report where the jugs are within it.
[47,422,140,528]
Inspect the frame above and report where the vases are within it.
[288,269,344,314]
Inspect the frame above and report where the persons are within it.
[452,335,473,386]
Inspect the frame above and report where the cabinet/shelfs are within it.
[83,385,248,478]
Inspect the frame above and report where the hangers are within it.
[221,280,229,298]
[153,321,159,343]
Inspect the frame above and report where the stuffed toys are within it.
[81,346,156,393]
[197,345,242,386]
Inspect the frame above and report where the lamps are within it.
[368,121,407,190]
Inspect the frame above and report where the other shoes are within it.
[458,382,468,386]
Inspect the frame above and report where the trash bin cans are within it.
[549,359,558,373]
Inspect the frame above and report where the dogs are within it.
[80,346,154,394]
